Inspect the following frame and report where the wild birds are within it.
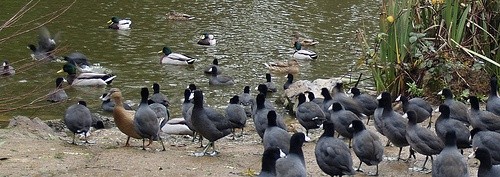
[0,10,500,177]
